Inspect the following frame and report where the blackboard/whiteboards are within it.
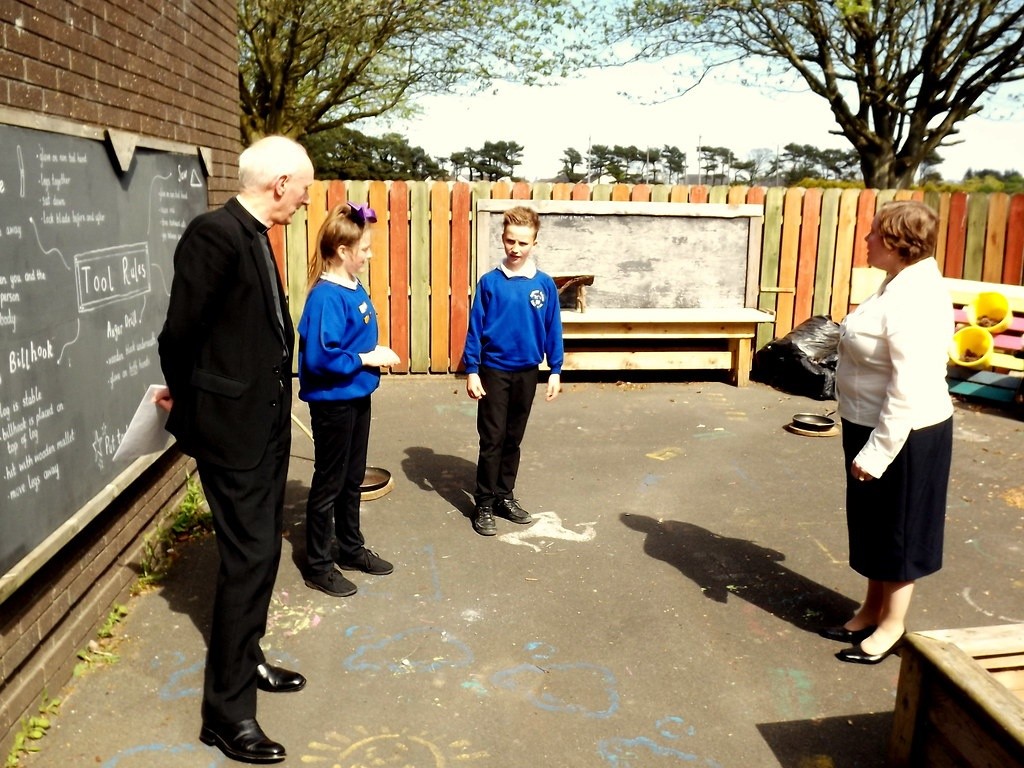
[0,104,213,602]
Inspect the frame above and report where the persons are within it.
[827,201,954,665]
[297,202,401,597]
[461,208,564,535]
[150,136,315,763]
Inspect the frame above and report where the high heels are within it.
[841,624,907,664]
[820,615,878,643]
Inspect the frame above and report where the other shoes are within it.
[305,563,357,597]
[339,546,394,575]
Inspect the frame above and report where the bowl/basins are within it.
[359,466,391,492]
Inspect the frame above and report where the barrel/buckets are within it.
[948,325,994,369]
[967,292,1013,333]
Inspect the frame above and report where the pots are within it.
[792,409,836,431]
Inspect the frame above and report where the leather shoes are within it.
[492,497,532,524]
[199,717,286,764]
[475,503,497,535]
[255,663,306,693]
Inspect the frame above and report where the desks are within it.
[538,307,778,387]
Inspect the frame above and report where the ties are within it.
[259,232,284,331]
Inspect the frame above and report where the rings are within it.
[859,478,864,481]
